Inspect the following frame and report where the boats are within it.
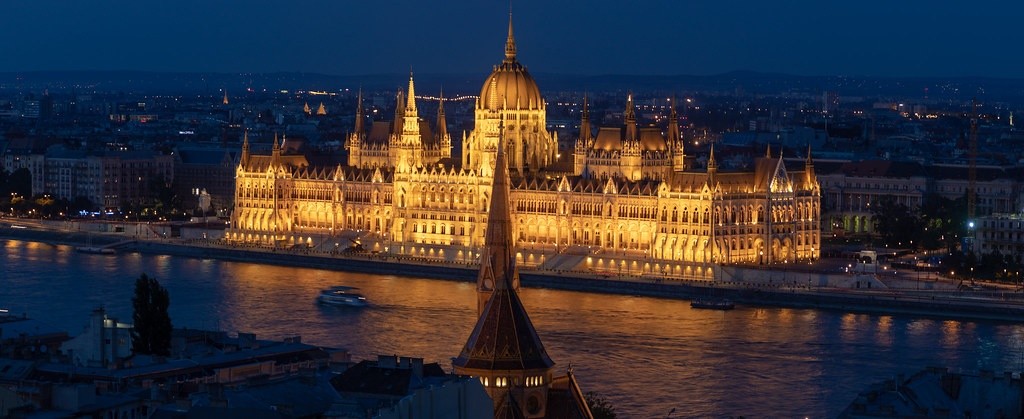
[76,246,114,254]
[316,285,370,309]
[689,298,735,310]
